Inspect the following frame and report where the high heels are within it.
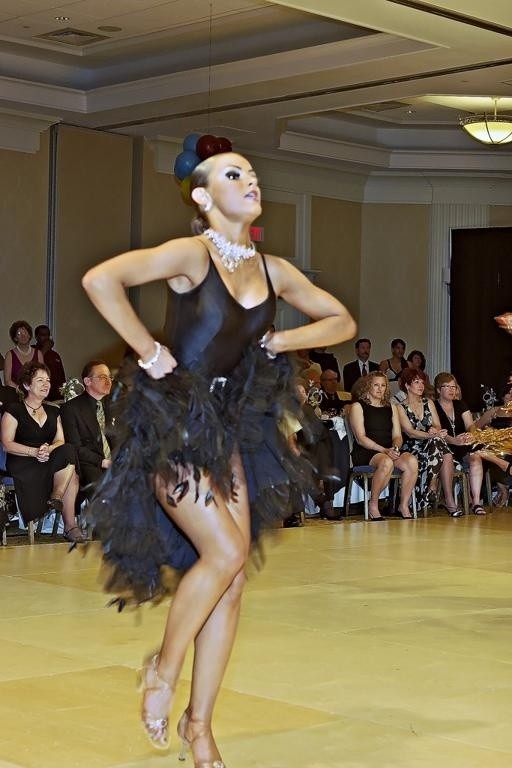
[398,509,413,519]
[444,505,463,516]
[368,512,385,521]
[177,705,225,768]
[137,652,175,751]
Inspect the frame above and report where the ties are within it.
[96,401,111,459]
[362,364,367,376]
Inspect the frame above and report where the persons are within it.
[69,131,359,768]
[2,321,132,542]
[279,338,512,529]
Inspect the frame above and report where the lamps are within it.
[454,99,512,146]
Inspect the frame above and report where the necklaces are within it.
[204,229,257,274]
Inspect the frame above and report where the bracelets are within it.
[132,341,160,369]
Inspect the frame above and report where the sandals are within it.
[63,527,86,542]
[471,504,487,515]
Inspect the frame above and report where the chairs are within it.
[344,404,402,526]
[5,471,61,546]
[436,465,494,518]
